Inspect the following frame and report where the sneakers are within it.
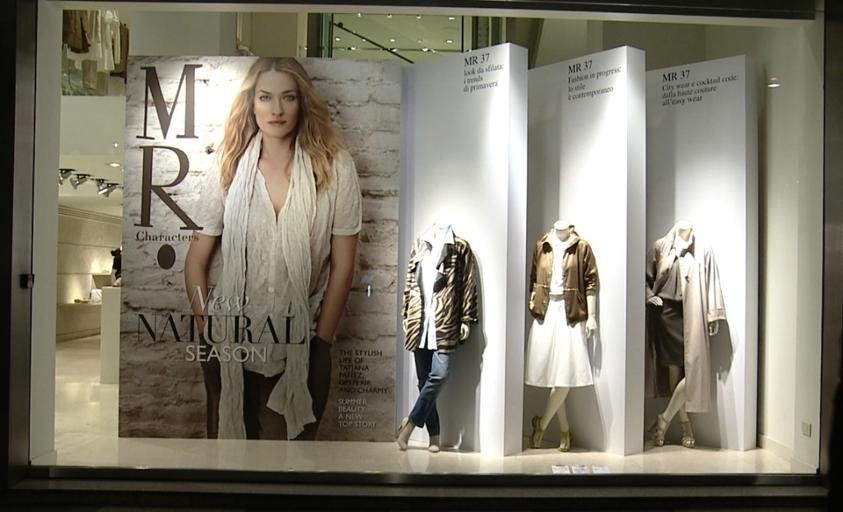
[559,430,571,451]
[531,415,547,449]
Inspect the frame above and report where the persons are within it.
[109,245,122,287]
[183,58,362,443]
[394,219,478,453]
[646,221,728,448]
[524,219,598,452]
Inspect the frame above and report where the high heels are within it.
[397,416,415,450]
[428,434,440,452]
[680,420,695,447]
[646,413,671,446]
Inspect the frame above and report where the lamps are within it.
[59,169,119,198]
